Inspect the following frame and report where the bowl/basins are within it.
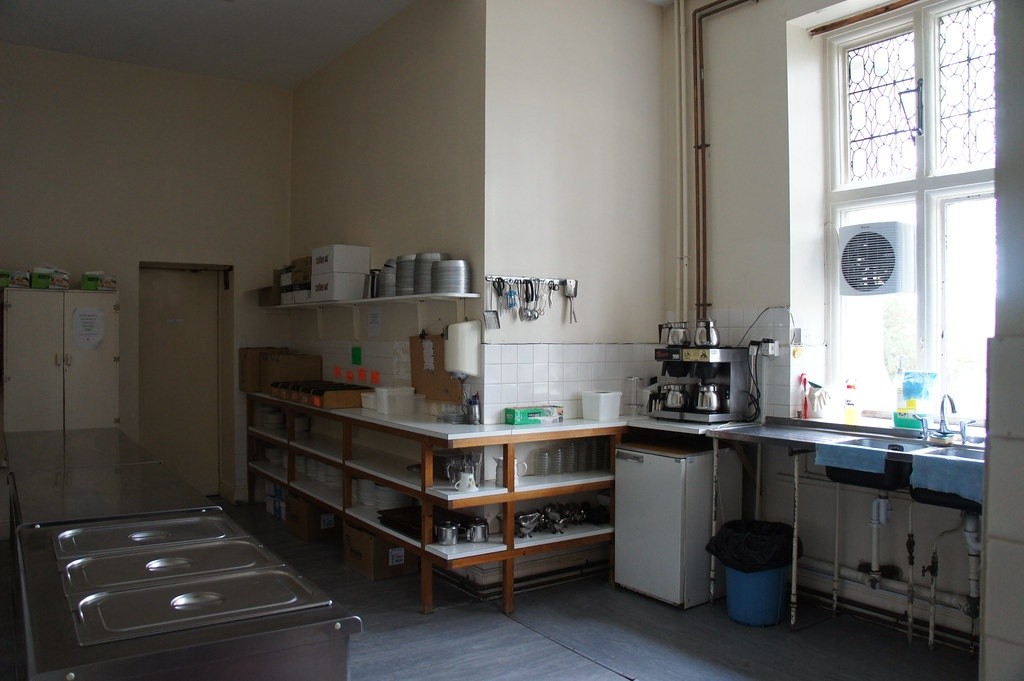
[380,252,471,296]
[295,417,308,431]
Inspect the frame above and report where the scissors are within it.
[493,277,505,317]
[548,281,560,307]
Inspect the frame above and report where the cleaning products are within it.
[844,376,862,426]
[894,355,916,419]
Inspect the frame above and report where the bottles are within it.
[844,386,856,423]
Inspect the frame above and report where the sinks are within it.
[823,438,932,492]
[910,446,985,516]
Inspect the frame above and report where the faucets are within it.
[937,393,957,434]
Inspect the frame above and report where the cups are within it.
[363,275,371,298]
[371,273,379,297]
[455,473,475,492]
[535,438,610,476]
[462,405,481,424]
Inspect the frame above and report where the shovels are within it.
[483,276,500,329]
[564,278,579,325]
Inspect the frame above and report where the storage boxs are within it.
[265,479,287,520]
[311,243,371,275]
[581,391,622,420]
[258,256,312,306]
[285,495,341,542]
[0,266,120,290]
[342,520,420,582]
[237,347,426,415]
[310,272,365,302]
[506,404,563,424]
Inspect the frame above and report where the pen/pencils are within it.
[463,392,479,405]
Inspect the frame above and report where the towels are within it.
[908,452,984,503]
[814,441,892,473]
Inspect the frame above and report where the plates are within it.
[352,478,404,510]
[295,454,342,486]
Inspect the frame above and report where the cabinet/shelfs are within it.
[0,287,121,429]
[978,334,1024,681]
[13,508,363,681]
[243,389,628,615]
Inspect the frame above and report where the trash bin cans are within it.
[720,519,802,628]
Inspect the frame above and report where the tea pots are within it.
[433,521,458,545]
[467,517,489,542]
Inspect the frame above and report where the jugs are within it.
[659,321,691,347]
[694,318,721,347]
[493,457,527,486]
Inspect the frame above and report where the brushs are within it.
[800,373,808,419]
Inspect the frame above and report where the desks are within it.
[2,428,216,573]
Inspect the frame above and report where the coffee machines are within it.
[683,348,749,421]
[649,348,694,420]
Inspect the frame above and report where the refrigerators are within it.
[614,443,743,610]
